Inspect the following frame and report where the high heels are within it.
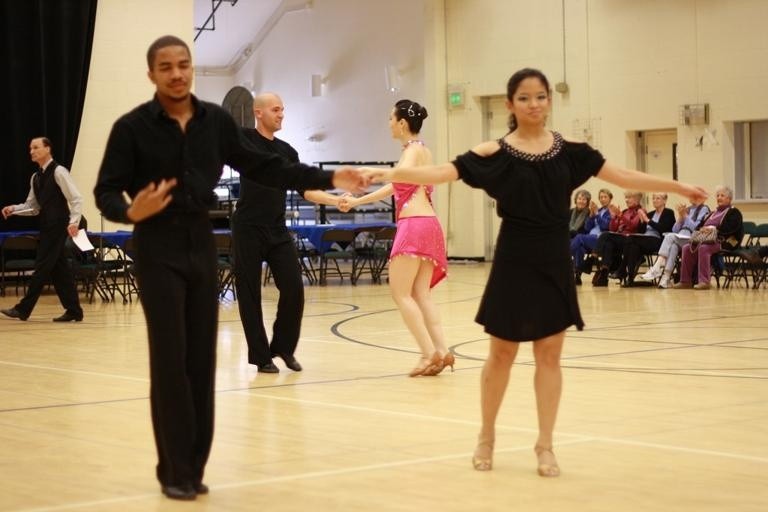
[410,351,443,378]
[423,352,454,375]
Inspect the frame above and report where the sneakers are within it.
[573,259,710,289]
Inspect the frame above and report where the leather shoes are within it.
[162,486,195,500]
[195,484,208,494]
[1,308,27,321]
[53,312,82,321]
[272,353,301,372]
[251,363,279,373]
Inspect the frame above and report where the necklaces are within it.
[399,138,425,150]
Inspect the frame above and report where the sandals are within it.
[535,444,560,476]
[473,435,495,470]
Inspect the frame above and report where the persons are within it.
[1,135,87,324]
[341,98,458,378]
[357,66,708,477]
[92,34,367,501]
[569,185,745,291]
[230,90,346,375]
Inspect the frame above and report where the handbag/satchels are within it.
[689,226,717,253]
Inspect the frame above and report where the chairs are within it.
[715,219,768,289]
